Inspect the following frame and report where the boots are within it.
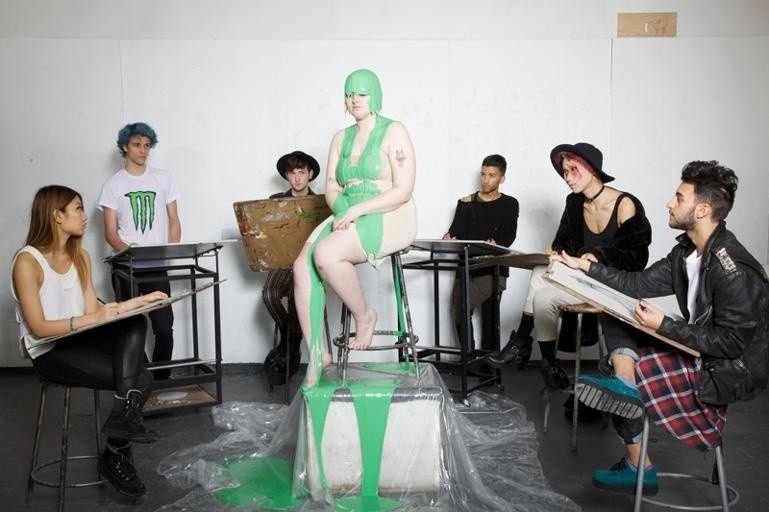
[96,442,148,499]
[102,389,162,447]
[484,330,534,373]
[538,358,574,401]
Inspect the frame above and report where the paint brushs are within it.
[96,297,120,315]
[635,289,648,312]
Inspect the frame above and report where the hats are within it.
[276,150,320,182]
[550,142,615,184]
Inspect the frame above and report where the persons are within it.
[6,182,159,501]
[287,67,421,393]
[93,120,185,389]
[546,159,769,499]
[438,151,524,379]
[482,141,655,391]
[257,149,322,387]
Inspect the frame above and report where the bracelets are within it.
[69,315,77,332]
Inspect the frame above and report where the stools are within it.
[268,287,332,406]
[332,245,422,390]
[631,409,742,512]
[24,372,122,507]
[540,302,610,454]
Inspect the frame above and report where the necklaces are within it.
[581,185,606,204]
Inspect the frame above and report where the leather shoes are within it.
[269,353,301,374]
[591,457,658,496]
[264,344,282,367]
[575,370,644,420]
